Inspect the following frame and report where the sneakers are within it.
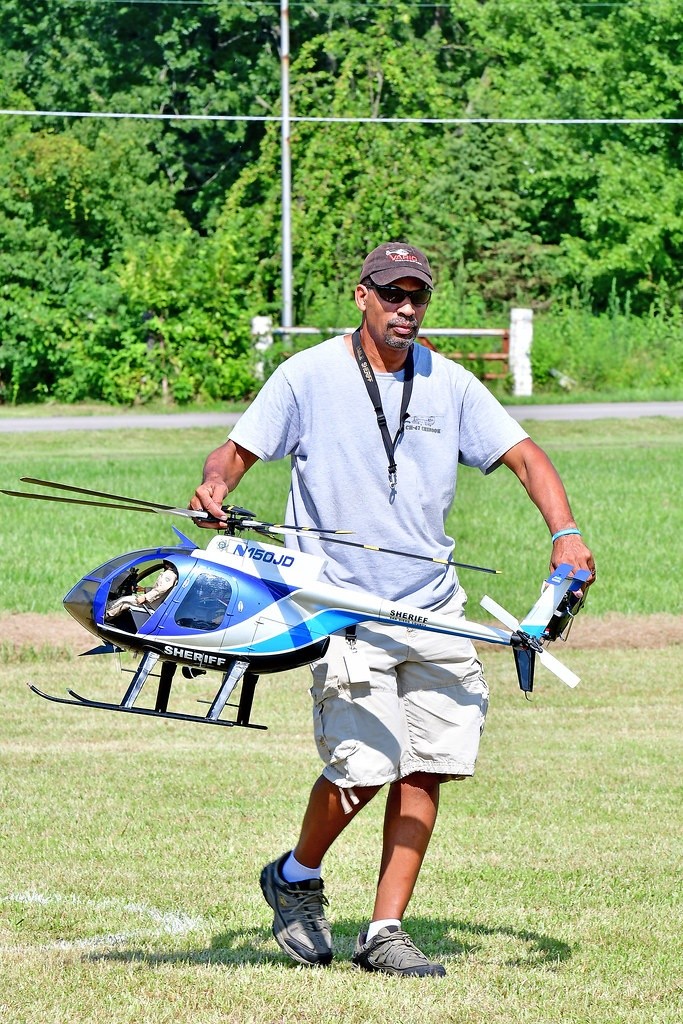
[259,849,334,967]
[350,925,446,978]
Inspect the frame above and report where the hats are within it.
[359,242,434,290]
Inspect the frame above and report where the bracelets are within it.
[552,529,581,543]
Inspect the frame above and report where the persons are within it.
[187,240,599,977]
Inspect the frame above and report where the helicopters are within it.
[0,477,592,731]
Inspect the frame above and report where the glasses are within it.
[366,284,432,305]
